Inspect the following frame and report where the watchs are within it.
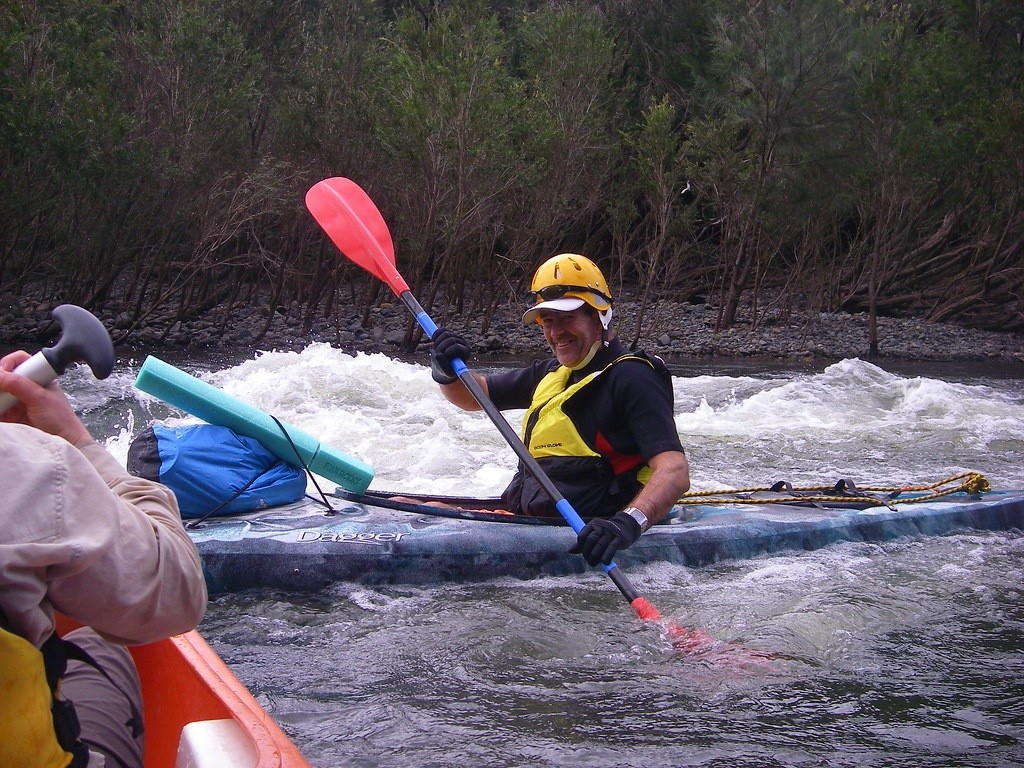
[622,507,648,533]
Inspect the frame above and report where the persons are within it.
[385,253,689,568]
[0,348,207,768]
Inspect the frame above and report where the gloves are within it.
[431,326,471,385]
[577,512,640,567]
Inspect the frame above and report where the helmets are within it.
[526,253,613,311]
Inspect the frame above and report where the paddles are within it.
[0,302,117,418]
[300,173,663,625]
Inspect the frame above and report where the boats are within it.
[54,613,307,768]
[178,472,1024,596]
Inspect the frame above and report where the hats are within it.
[521,298,612,332]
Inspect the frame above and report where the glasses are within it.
[526,284,611,306]
